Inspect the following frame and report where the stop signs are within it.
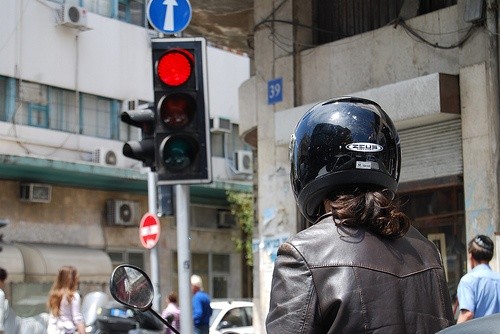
[139,212,161,249]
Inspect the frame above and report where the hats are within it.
[190,274,202,288]
[472,235,494,254]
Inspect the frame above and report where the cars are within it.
[209,301,254,334]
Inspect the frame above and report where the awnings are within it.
[0,240,113,288]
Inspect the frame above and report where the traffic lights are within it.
[119,103,156,171]
[150,38,212,185]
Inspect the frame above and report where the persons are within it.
[265,95,455,334]
[457,234,500,325]
[46,265,87,334]
[160,290,180,334]
[189,274,213,334]
[0,266,11,334]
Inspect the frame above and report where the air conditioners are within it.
[106,200,141,227]
[92,146,124,166]
[232,150,254,174]
[55,4,87,29]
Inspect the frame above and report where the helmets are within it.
[288,96,401,224]
